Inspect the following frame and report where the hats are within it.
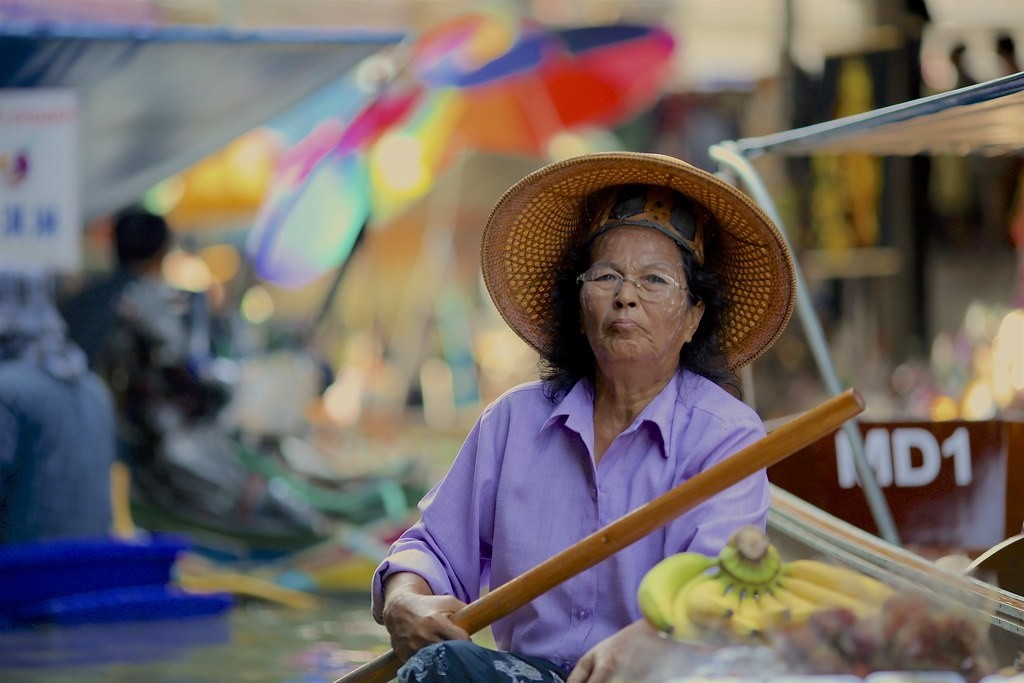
[478,150,796,376]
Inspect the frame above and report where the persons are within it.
[0,272,114,547]
[371,150,796,682]
[59,208,240,461]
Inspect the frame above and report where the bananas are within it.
[635,526,892,646]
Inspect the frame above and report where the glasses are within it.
[575,262,698,304]
